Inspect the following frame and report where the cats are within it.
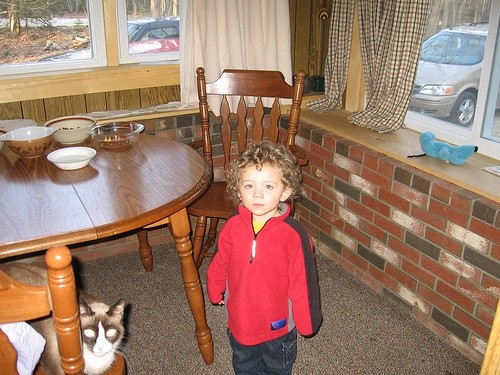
[0,260,127,375]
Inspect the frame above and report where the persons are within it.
[206,140,323,375]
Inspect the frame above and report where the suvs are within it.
[39,16,180,65]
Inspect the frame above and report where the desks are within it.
[0,138,215,366]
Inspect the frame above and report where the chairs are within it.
[0,245,126,375]
[186,67,309,268]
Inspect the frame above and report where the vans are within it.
[407,22,500,127]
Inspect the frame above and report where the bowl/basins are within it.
[87,121,144,151]
[46,147,96,171]
[0,126,61,159]
[43,115,97,144]
